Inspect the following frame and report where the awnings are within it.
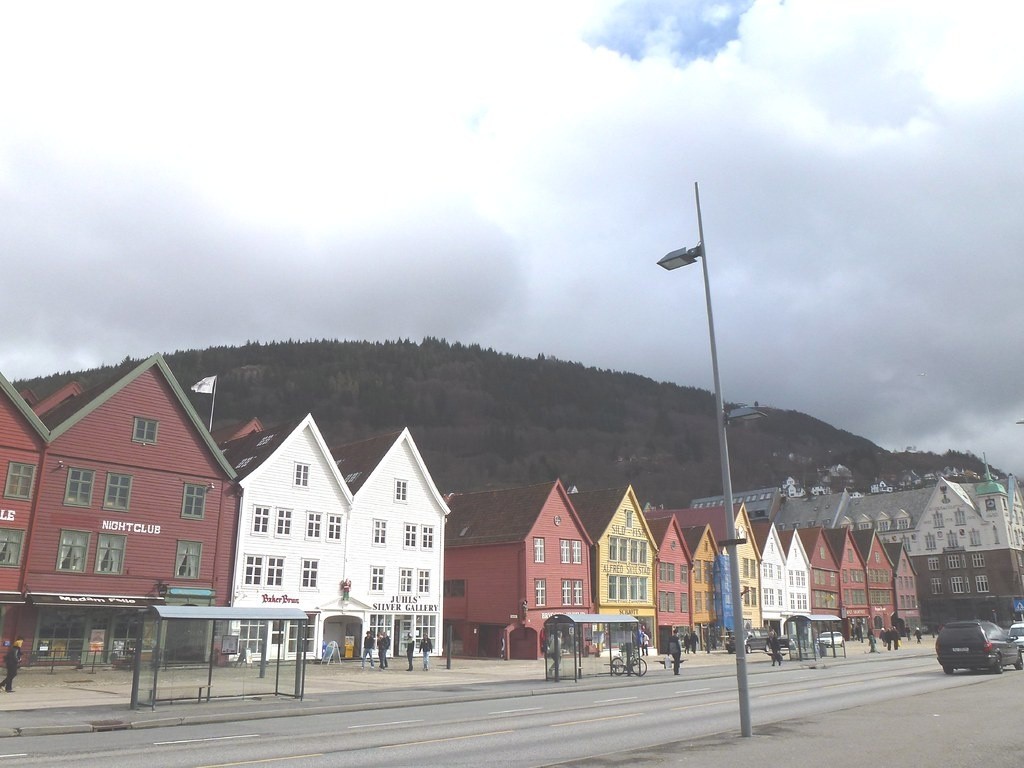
[25,593,165,609]
[0,592,26,604]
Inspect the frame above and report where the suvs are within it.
[935,619,1024,675]
[816,631,845,647]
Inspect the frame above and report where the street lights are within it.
[655,183,766,736]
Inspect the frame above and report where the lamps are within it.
[156,582,169,595]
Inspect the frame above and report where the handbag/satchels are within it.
[897,639,901,647]
[664,657,672,669]
[777,653,782,660]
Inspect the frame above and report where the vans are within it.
[725,629,771,654]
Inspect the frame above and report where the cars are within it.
[778,634,808,655]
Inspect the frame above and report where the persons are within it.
[852,624,863,643]
[668,629,681,675]
[642,632,649,656]
[768,631,781,666]
[378,632,390,670]
[548,631,562,671]
[868,627,876,645]
[879,626,901,650]
[903,624,911,641]
[419,633,432,670]
[684,634,690,654]
[869,635,876,653]
[771,629,777,637]
[0,636,24,694]
[406,632,415,671]
[690,631,698,654]
[914,627,922,644]
[360,631,375,669]
[929,621,944,638]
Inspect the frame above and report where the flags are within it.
[191,375,216,394]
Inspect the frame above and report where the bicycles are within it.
[611,651,647,677]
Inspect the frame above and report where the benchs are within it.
[148,685,213,705]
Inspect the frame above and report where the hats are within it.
[366,631,372,635]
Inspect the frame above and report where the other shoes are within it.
[406,668,413,672]
[378,667,384,671]
[370,666,375,669]
[423,668,429,672]
[675,673,681,676]
[360,666,364,670]
[6,690,14,694]
[1,682,4,690]
[384,666,389,670]
